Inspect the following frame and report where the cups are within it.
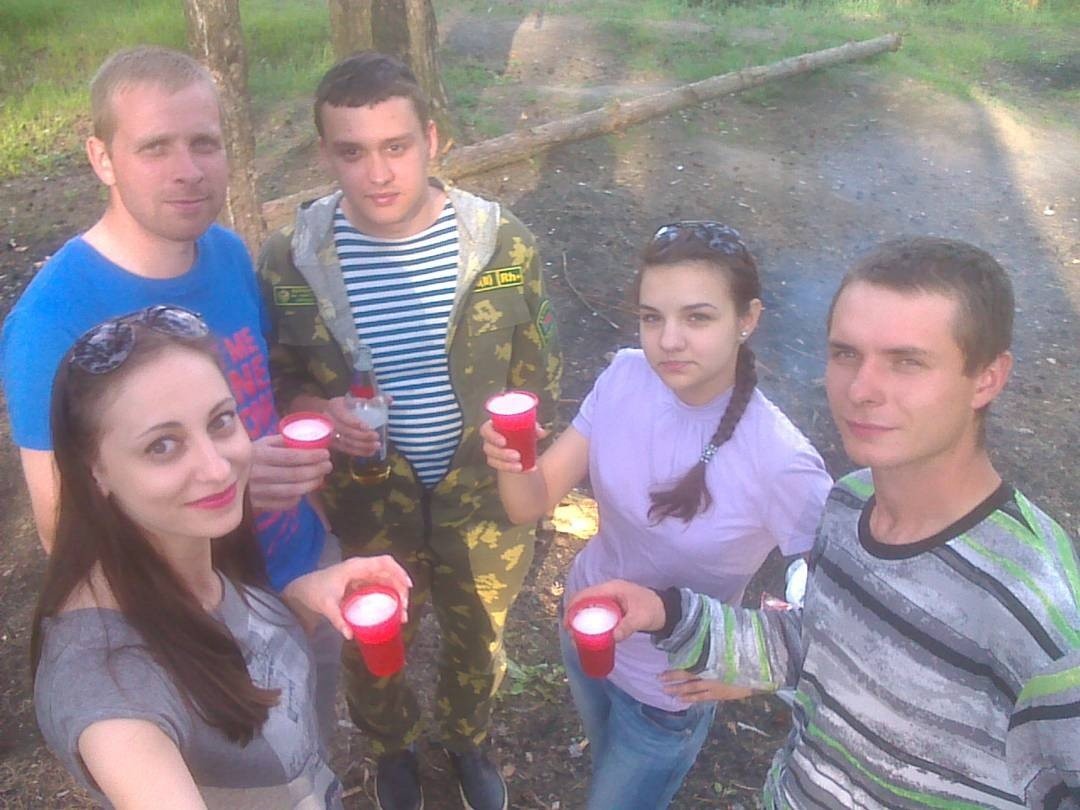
[340,586,404,675]
[565,594,622,675]
[276,412,334,457]
[483,389,541,474]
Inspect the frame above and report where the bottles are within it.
[340,344,390,487]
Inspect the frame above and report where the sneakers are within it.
[443,743,508,810]
[372,747,425,810]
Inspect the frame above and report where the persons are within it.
[0,44,343,721]
[30,305,413,810]
[480,221,833,810]
[565,234,1080,810]
[254,51,564,810]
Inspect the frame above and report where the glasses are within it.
[66,307,210,376]
[647,220,748,257]
